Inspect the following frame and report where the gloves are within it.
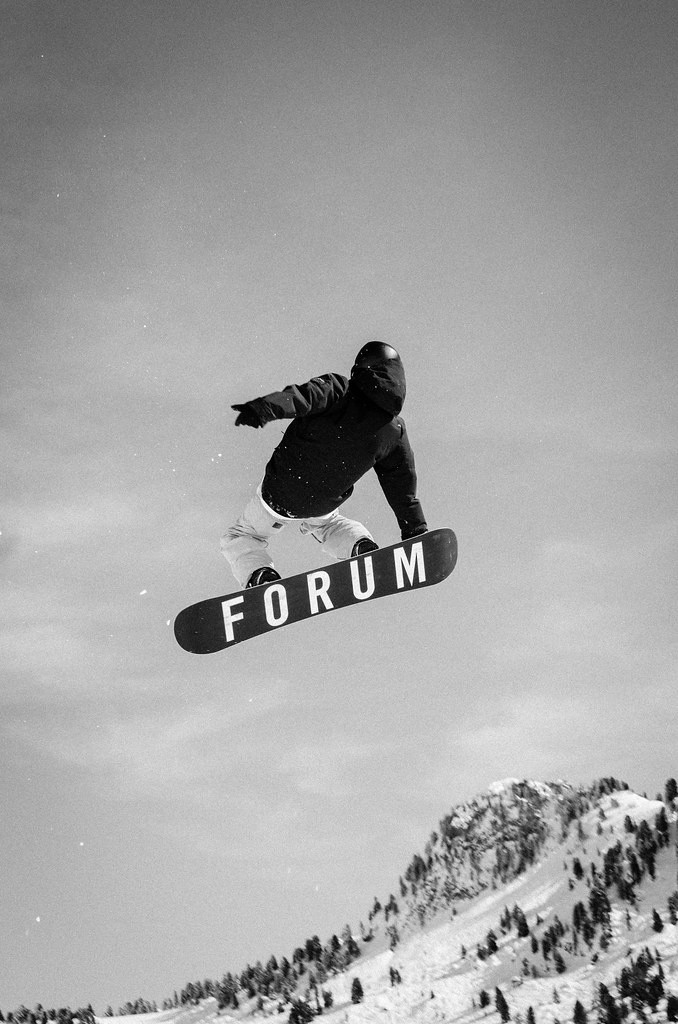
[231,403,260,429]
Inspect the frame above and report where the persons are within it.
[219,341,428,589]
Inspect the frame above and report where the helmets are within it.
[355,341,399,365]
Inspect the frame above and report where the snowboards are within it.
[173,527,460,656]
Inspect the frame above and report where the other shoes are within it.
[357,541,376,553]
[256,570,277,585]
